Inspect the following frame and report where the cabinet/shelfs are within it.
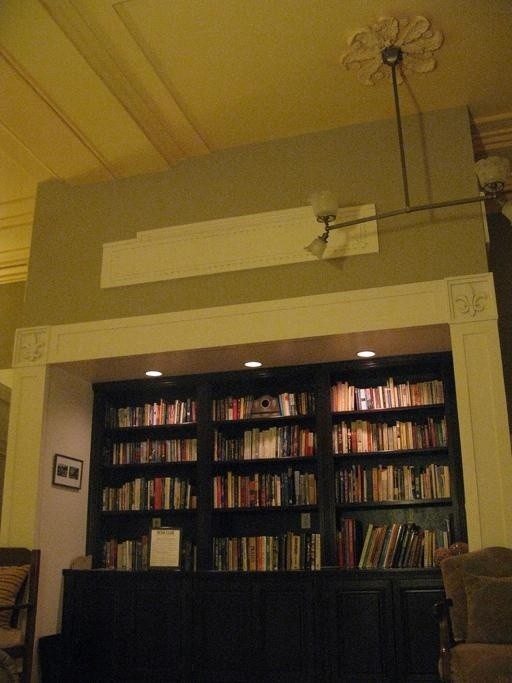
[59,352,462,682]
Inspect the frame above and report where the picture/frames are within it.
[51,452,82,490]
[147,526,183,572]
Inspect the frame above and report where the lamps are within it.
[300,10,510,261]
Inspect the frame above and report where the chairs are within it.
[0,545,41,683]
[430,544,511,682]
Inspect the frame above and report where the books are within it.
[330,378,448,412]
[103,477,197,511]
[332,416,449,454]
[337,519,451,568]
[213,392,316,422]
[214,467,316,508]
[105,398,196,429]
[211,531,321,568]
[113,439,197,464]
[214,425,317,461]
[102,536,197,568]
[335,464,451,503]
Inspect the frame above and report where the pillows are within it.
[459,570,511,643]
[0,563,31,631]
